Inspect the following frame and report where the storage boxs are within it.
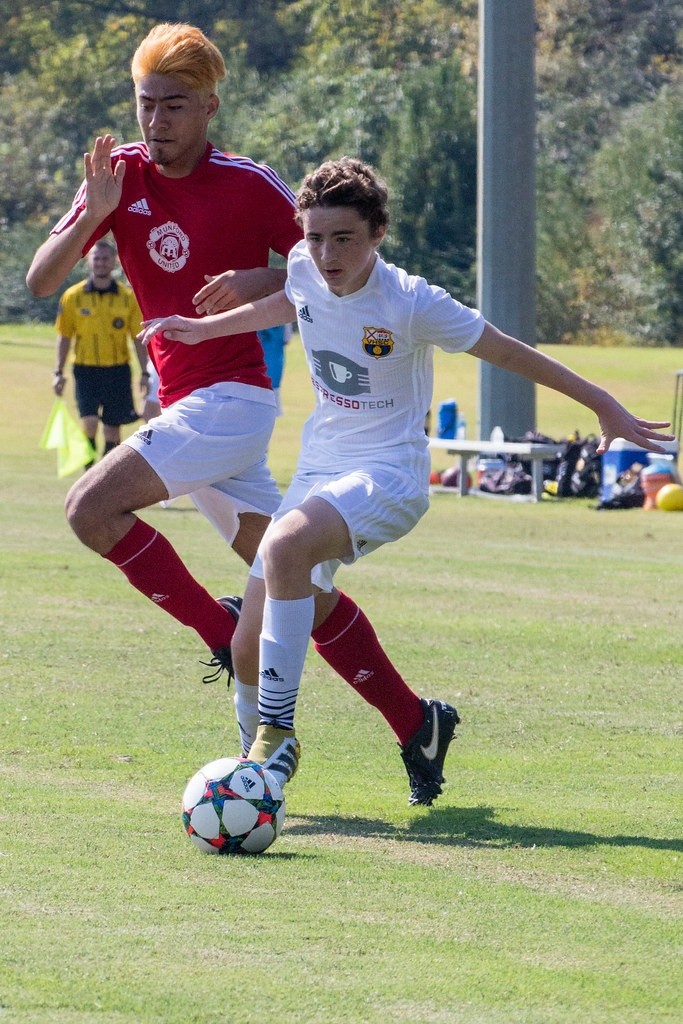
[602,436,680,503]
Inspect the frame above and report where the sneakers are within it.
[396,697,461,808]
[198,595,243,692]
[246,719,302,792]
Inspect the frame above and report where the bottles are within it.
[457,413,467,439]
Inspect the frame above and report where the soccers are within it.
[180,753,287,859]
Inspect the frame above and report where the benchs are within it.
[427,440,569,502]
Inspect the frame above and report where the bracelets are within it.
[142,372,150,377]
[55,370,62,375]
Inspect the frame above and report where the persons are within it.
[143,361,162,422]
[256,323,292,416]
[135,157,677,791]
[53,241,151,471]
[25,22,462,806]
